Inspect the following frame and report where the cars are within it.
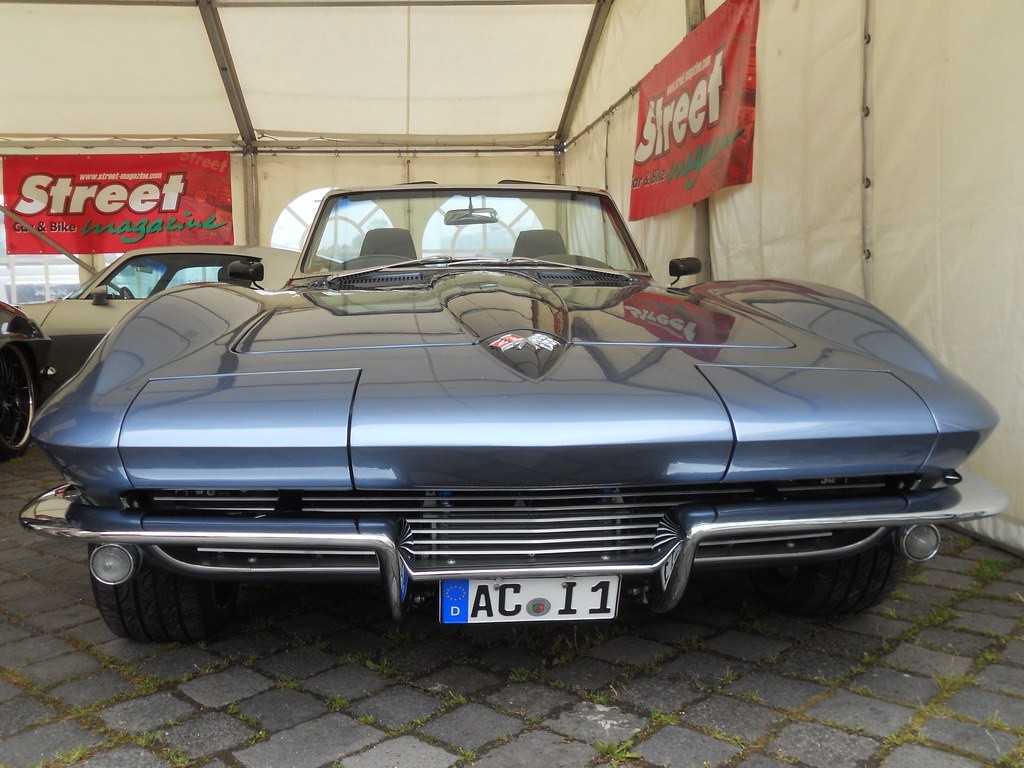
[0,302,58,462]
[13,245,343,337]
[17,180,1011,646]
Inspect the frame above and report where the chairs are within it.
[512,228,566,260]
[359,227,417,270]
